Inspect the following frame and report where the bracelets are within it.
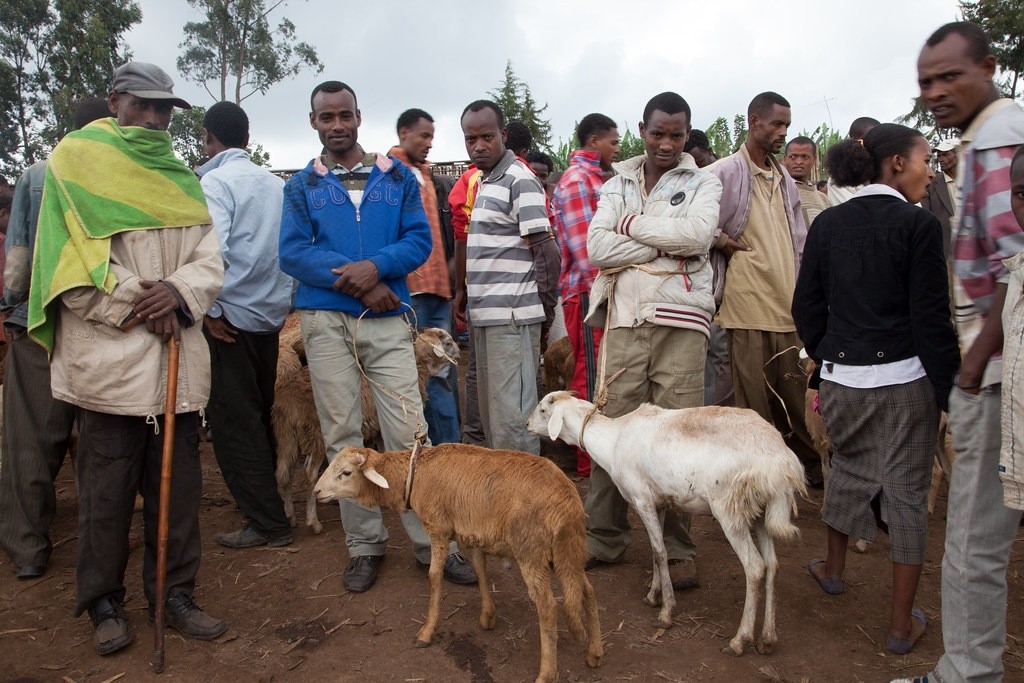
[956,384,980,389]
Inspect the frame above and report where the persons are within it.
[998,143,1024,511]
[388,108,460,445]
[702,92,808,489]
[576,92,723,590]
[28,62,228,656]
[192,157,211,179]
[848,117,881,141]
[550,94,619,479]
[278,81,480,592]
[0,97,119,578]
[889,21,1024,683]
[778,136,833,234]
[191,101,302,549]
[791,123,960,655]
[449,98,557,456]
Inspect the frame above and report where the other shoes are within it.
[16,565,43,577]
[464,430,487,447]
[810,557,843,594]
[799,460,823,486]
[887,609,926,655]
[890,676,928,683]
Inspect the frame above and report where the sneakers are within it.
[583,552,609,570]
[668,559,696,589]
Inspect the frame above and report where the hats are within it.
[112,62,193,109]
[930,139,961,152]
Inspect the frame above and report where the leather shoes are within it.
[146,592,228,639]
[416,551,479,584]
[215,523,294,549]
[91,599,132,655]
[342,555,385,592]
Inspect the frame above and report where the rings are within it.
[390,294,395,298]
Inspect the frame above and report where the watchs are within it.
[206,302,223,319]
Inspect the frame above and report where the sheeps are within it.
[271,309,308,389]
[313,443,603,683]
[544,336,576,390]
[799,347,950,553]
[270,327,459,535]
[523,391,808,658]
